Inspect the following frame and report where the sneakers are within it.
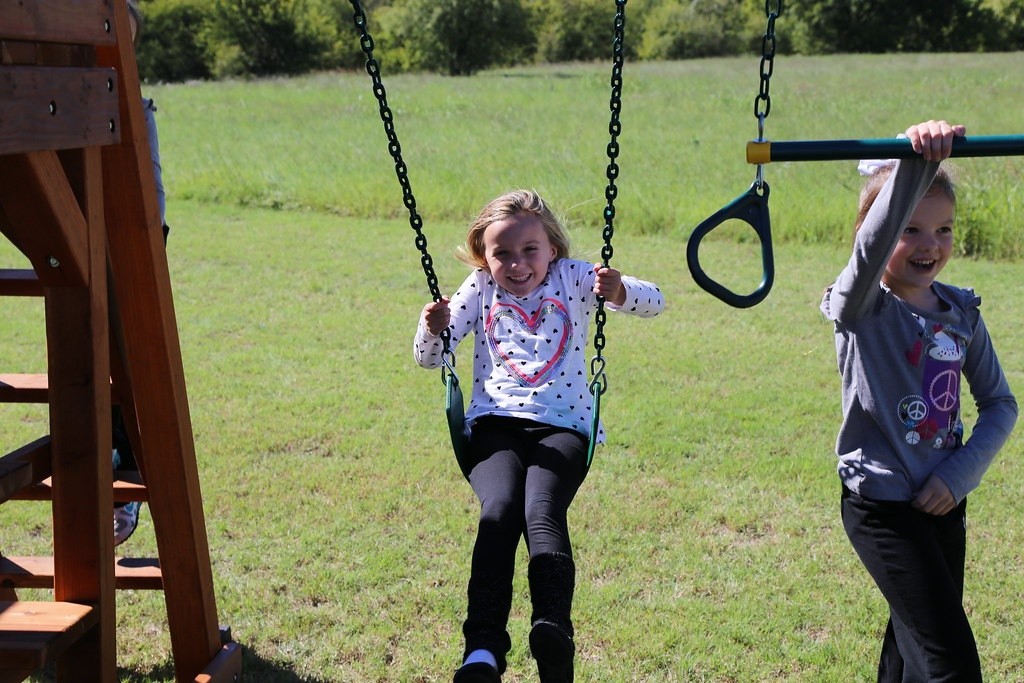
[114,501,142,546]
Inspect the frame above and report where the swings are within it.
[353,0,627,484]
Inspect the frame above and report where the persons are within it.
[823,117,1020,683]
[412,190,663,683]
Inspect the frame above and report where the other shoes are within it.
[454,662,501,683]
[529,619,575,683]
